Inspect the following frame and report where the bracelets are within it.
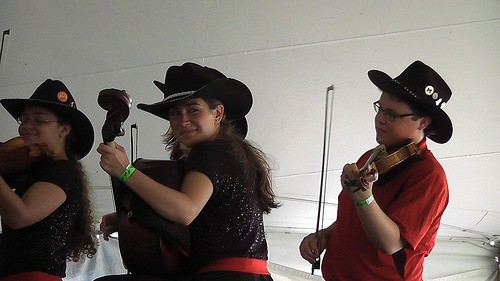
[117,163,136,182]
[353,195,374,208]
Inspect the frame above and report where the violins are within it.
[351,140,419,194]
[0,134,55,196]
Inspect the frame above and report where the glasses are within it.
[17,116,57,125]
[373,100,414,121]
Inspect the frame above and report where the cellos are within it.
[96,86,191,279]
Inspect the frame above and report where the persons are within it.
[299,60,452,281]
[96,62,281,281]
[0,78,95,281]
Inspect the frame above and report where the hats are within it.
[0,79,95,161]
[136,62,253,140]
[368,60,453,144]
